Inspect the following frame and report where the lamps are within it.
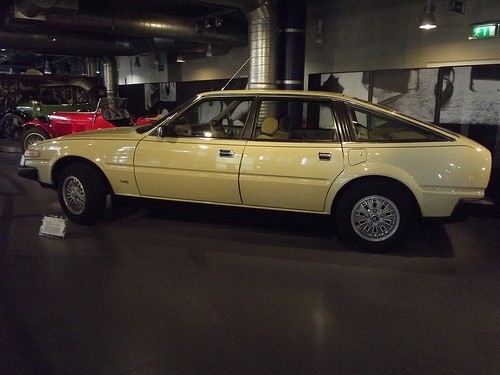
[206,45,212,58]
[313,19,326,44]
[176,52,186,62]
[153,53,161,64]
[134,56,141,68]
[418,0,438,30]
[42,56,52,74]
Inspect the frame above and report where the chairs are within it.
[272,116,293,139]
[255,117,279,139]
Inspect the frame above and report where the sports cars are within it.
[18,95,189,157]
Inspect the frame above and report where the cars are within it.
[15,88,493,254]
[0,75,125,142]
